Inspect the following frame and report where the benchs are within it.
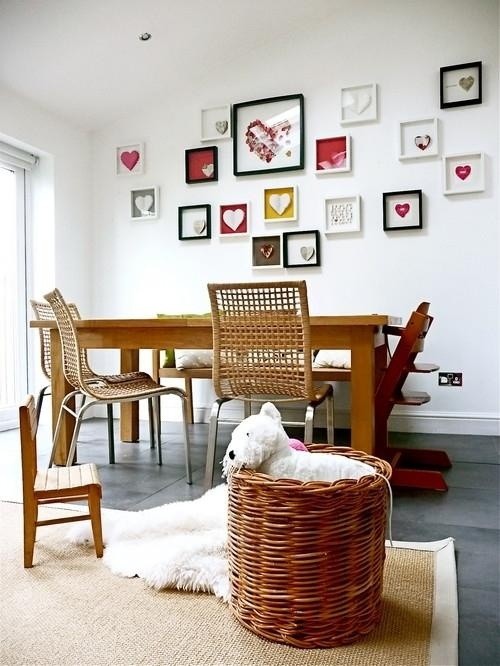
[150,349,351,433]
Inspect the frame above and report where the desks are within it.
[28,314,388,467]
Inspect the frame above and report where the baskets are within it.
[227,443,393,650]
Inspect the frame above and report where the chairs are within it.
[47,286,189,486]
[374,301,454,493]
[31,301,155,465]
[205,281,334,489]
[18,394,104,568]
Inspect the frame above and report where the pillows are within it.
[156,308,356,370]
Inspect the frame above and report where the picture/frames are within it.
[382,190,423,230]
[127,185,157,221]
[442,151,489,196]
[438,60,482,109]
[396,116,440,160]
[177,81,376,268]
[115,143,144,178]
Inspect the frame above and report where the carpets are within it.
[0,493,460,666]
